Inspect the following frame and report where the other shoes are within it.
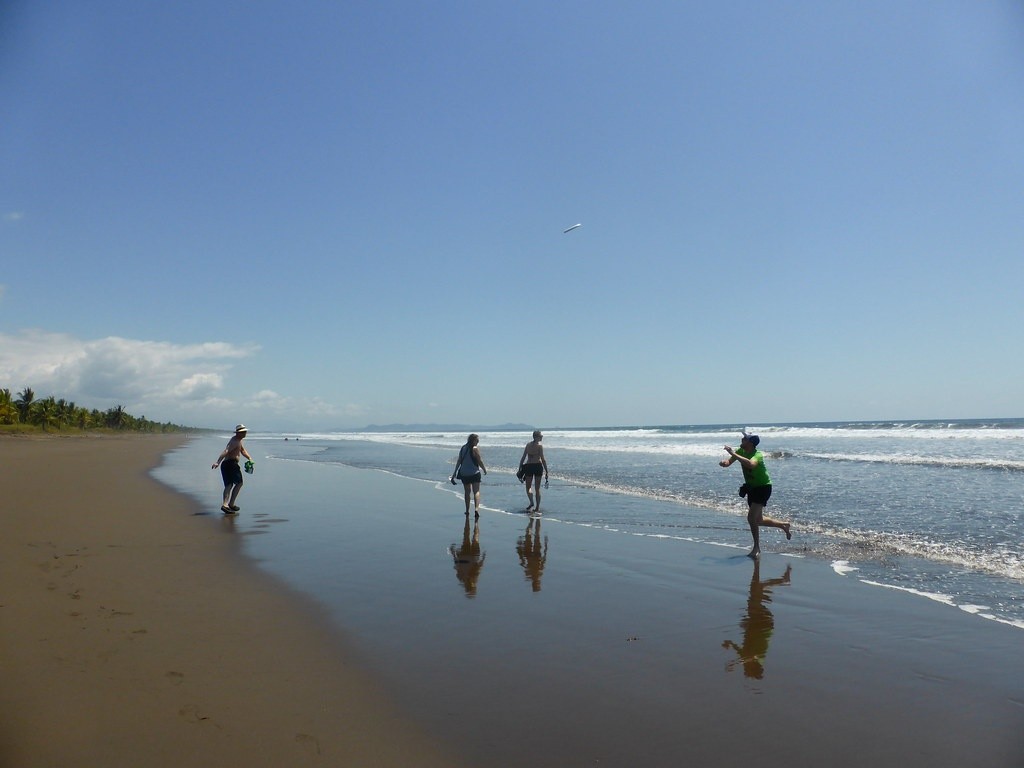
[228,504,240,511]
[221,506,235,514]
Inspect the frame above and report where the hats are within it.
[742,431,760,445]
[234,424,249,433]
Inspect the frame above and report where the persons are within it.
[518,430,548,513]
[450,434,488,517]
[212,423,250,513]
[719,435,792,557]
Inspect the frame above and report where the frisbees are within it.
[564,223,580,233]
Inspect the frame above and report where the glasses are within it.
[536,435,543,438]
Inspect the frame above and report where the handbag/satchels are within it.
[457,467,462,479]
